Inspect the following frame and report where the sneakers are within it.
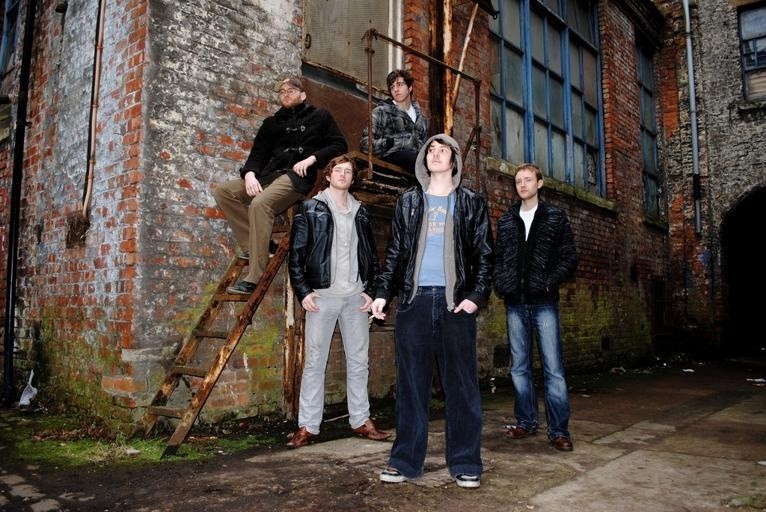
[237,243,278,260]
[379,463,409,483]
[226,280,258,294]
[456,473,481,487]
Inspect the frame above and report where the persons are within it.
[215,78,348,295]
[286,156,391,449]
[371,135,495,489]
[492,163,579,452]
[359,69,427,173]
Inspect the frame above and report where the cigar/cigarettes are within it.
[369,315,374,319]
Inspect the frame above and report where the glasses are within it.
[278,88,302,94]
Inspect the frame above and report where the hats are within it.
[275,77,305,91]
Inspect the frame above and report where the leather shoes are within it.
[506,426,537,438]
[287,426,319,448]
[352,420,391,440]
[552,436,573,452]
